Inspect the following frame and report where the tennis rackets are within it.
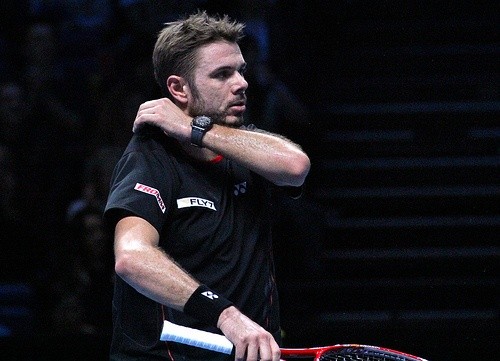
[158,319,431,361]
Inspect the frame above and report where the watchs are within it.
[190,114,214,149]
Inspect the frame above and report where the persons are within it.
[1,0,155,361]
[235,31,312,135]
[101,9,312,361]
